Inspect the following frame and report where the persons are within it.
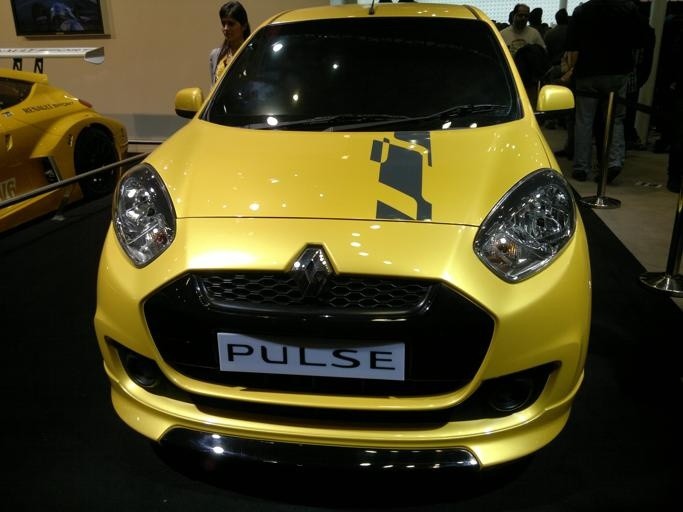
[209,0,252,87]
[48,2,76,32]
[499,0,656,185]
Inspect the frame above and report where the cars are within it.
[93,1,592,489]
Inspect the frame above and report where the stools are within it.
[578,73,632,209]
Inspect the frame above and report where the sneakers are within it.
[572,169,586,182]
[599,165,621,184]
